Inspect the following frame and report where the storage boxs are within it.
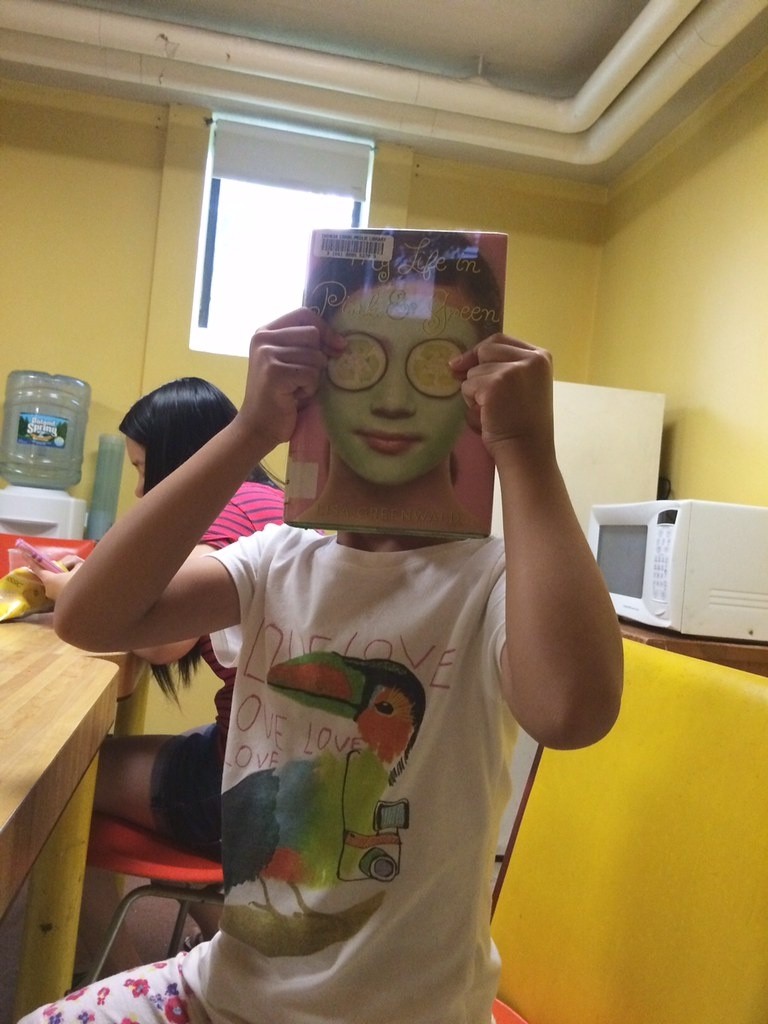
[0,533,97,581]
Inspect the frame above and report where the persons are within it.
[292,231,500,536]
[22,377,324,948]
[17,308,626,1022]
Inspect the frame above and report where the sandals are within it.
[183,933,203,952]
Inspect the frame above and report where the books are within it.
[283,228,509,538]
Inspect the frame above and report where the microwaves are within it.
[588,498,768,643]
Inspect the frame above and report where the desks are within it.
[0,610,150,1024]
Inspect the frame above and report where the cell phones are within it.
[15,538,65,574]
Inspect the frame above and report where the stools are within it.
[85,810,223,985]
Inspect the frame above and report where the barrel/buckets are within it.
[0,368,92,490]
